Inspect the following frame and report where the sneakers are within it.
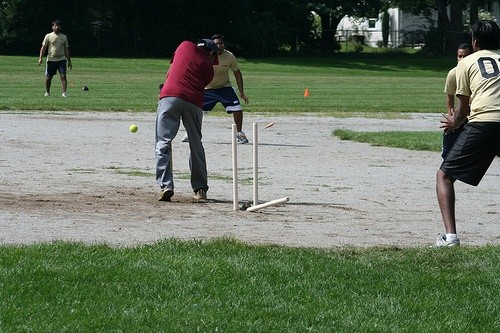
[436,234,460,247]
[181,133,188,141]
[158,187,174,202]
[191,190,208,202]
[237,132,248,144]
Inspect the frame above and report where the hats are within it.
[198,38,220,66]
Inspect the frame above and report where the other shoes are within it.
[62,92,68,97]
[45,91,51,96]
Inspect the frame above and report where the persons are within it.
[181,34,249,144]
[441,43,474,159]
[154,39,219,203]
[38,20,73,97]
[436,20,500,248]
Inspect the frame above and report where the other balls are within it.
[82,85,89,91]
[129,125,138,133]
